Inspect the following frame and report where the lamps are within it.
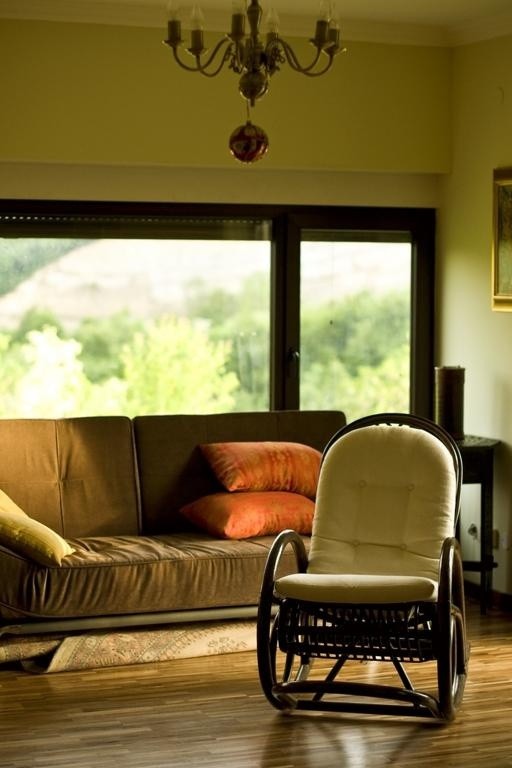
[160,1,350,165]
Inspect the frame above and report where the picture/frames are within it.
[487,163,512,315]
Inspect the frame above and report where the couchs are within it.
[0,406,351,678]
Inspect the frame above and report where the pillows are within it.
[193,438,326,504]
[175,487,317,542]
[0,488,78,570]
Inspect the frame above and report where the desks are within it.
[447,430,504,622]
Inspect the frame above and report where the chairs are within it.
[254,411,472,730]
